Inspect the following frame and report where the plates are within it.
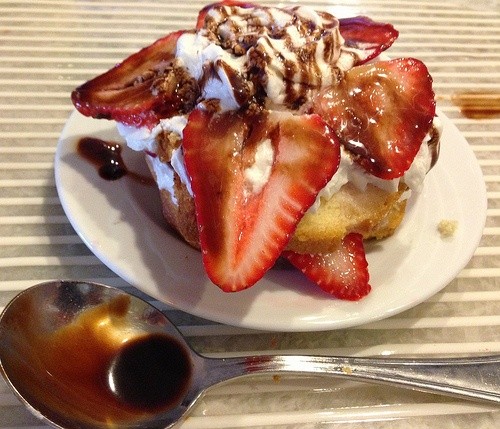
[54,51,487,332]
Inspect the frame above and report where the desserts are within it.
[69,0,439,302]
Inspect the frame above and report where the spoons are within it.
[1,280,500,429]
